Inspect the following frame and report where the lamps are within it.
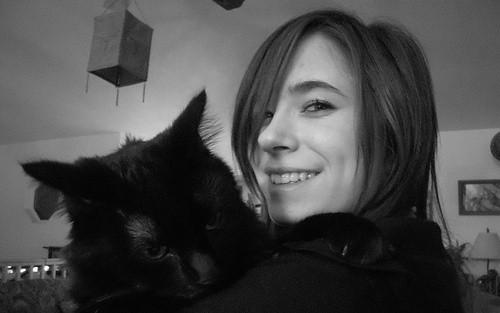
[469,228,500,273]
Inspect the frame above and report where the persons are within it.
[180,8,465,313]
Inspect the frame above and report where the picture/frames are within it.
[459,180,500,216]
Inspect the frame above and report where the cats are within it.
[16,88,397,313]
